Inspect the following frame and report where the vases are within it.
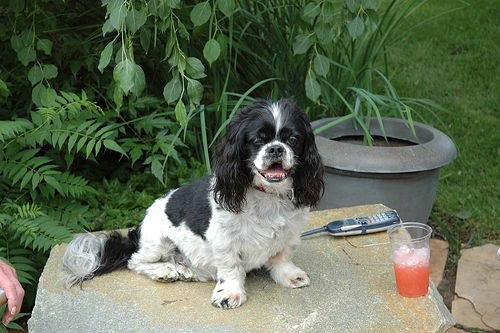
[310,118,457,239]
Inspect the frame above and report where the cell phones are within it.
[300,211,400,236]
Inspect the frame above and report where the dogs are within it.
[57,98,326,310]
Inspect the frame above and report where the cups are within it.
[387,222,432,297]
